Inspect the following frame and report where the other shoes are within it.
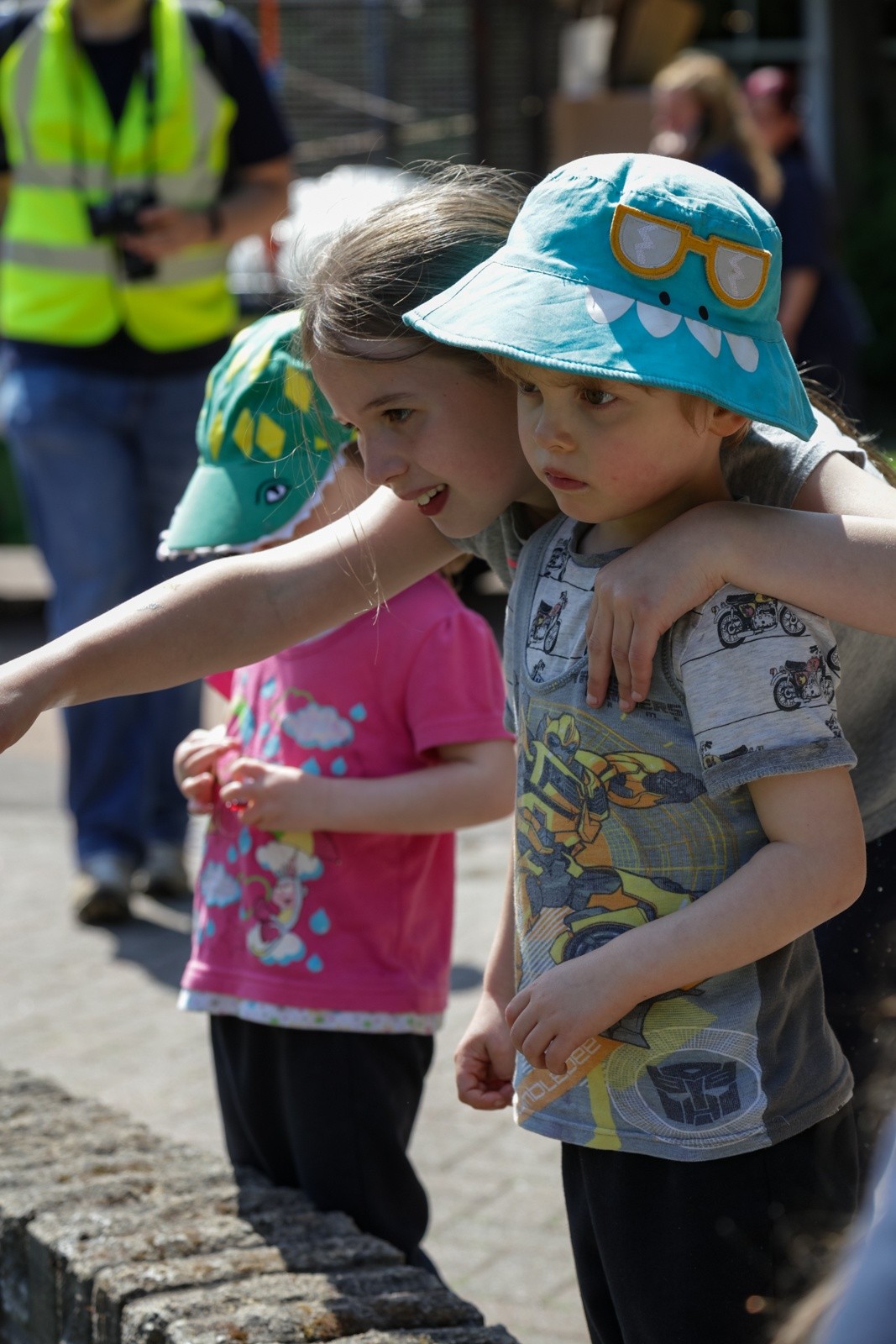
[76,880,135,928]
[142,865,191,902]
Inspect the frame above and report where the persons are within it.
[157,307,518,1278]
[0,0,294,937]
[648,52,823,350]
[0,159,896,1180]
[401,152,865,1344]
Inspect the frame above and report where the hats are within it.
[156,309,351,561]
[398,151,820,445]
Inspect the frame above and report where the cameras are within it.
[86,188,163,283]
